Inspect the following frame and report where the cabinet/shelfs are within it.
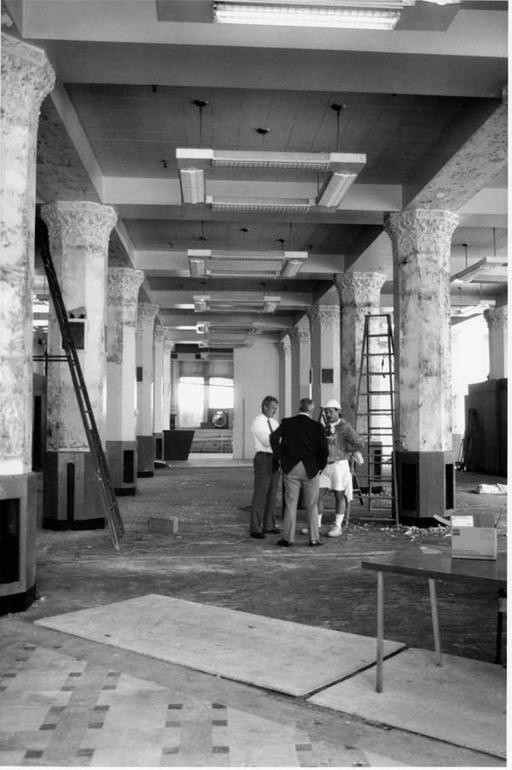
[175,428,232,453]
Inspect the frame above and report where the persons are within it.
[299,399,365,538]
[248,395,281,539]
[269,398,330,548]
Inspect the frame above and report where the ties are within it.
[268,420,273,433]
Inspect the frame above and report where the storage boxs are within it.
[432,512,504,562]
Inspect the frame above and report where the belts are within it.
[257,452,273,456]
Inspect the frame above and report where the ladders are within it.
[314,406,365,507]
[343,312,400,532]
[31,202,129,552]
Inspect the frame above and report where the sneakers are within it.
[300,528,309,535]
[327,526,343,537]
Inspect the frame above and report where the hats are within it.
[322,399,341,409]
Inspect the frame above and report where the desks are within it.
[361,544,506,693]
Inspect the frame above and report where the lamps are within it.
[175,98,214,206]
[192,282,211,313]
[311,101,368,215]
[450,227,508,287]
[210,3,404,31]
[185,222,212,281]
[279,222,309,281]
[193,321,213,363]
[258,280,281,316]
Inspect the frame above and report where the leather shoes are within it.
[278,539,293,547]
[309,540,323,546]
[263,527,280,534]
[250,532,265,539]
